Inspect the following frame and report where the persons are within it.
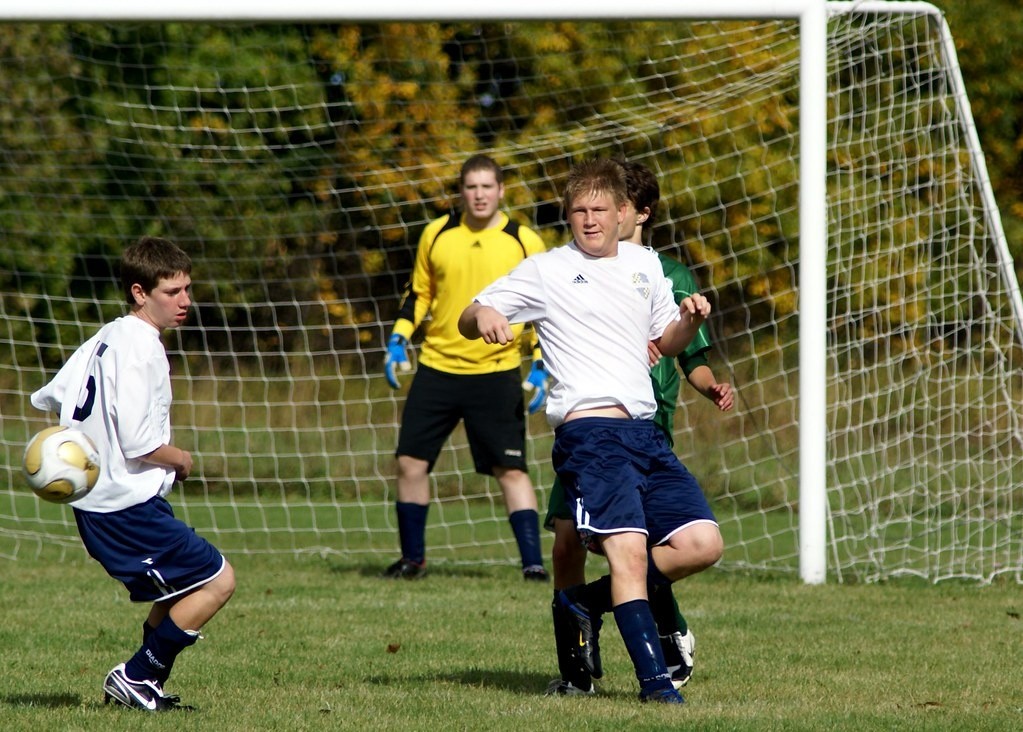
[458,158,735,704]
[30,236,236,712]
[386,155,550,581]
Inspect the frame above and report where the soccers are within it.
[21,423,103,506]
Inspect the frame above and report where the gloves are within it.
[521,360,548,416]
[382,334,411,389]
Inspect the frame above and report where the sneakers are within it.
[384,555,427,582]
[561,583,602,680]
[523,566,550,585]
[543,678,593,698]
[639,684,682,704]
[101,662,194,714]
[657,627,695,690]
